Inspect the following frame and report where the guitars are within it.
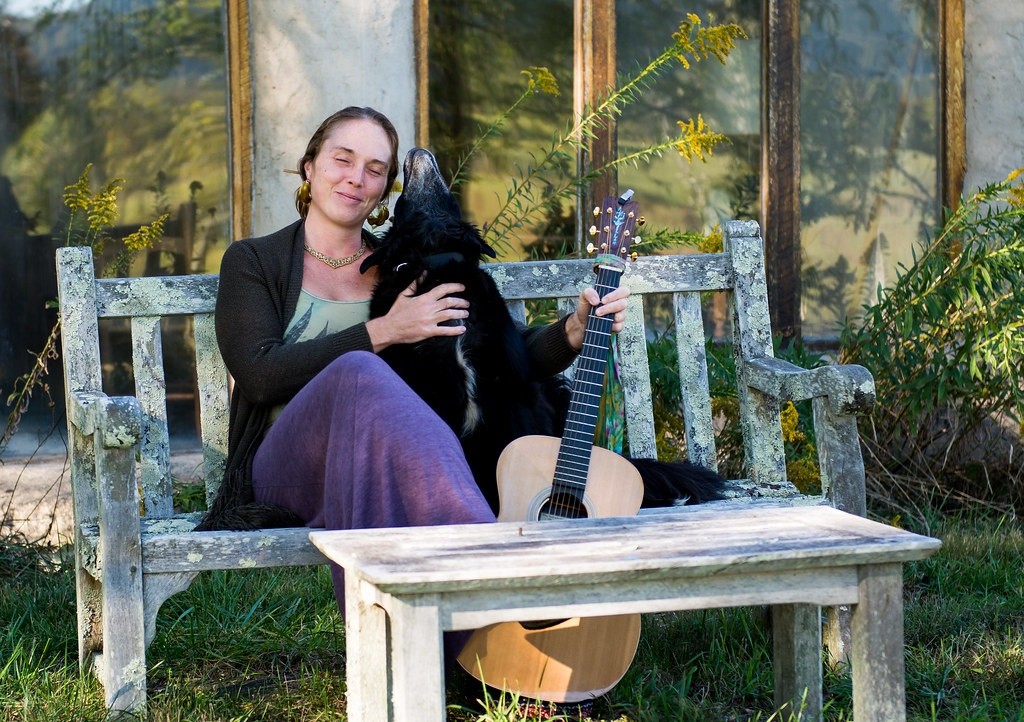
[453,188,647,709]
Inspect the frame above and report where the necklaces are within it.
[303,243,365,269]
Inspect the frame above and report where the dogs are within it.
[368,146,724,516]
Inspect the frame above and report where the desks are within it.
[307,505,942,722]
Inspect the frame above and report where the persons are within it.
[197,105,631,632]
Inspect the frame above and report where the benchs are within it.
[54,218,882,717]
